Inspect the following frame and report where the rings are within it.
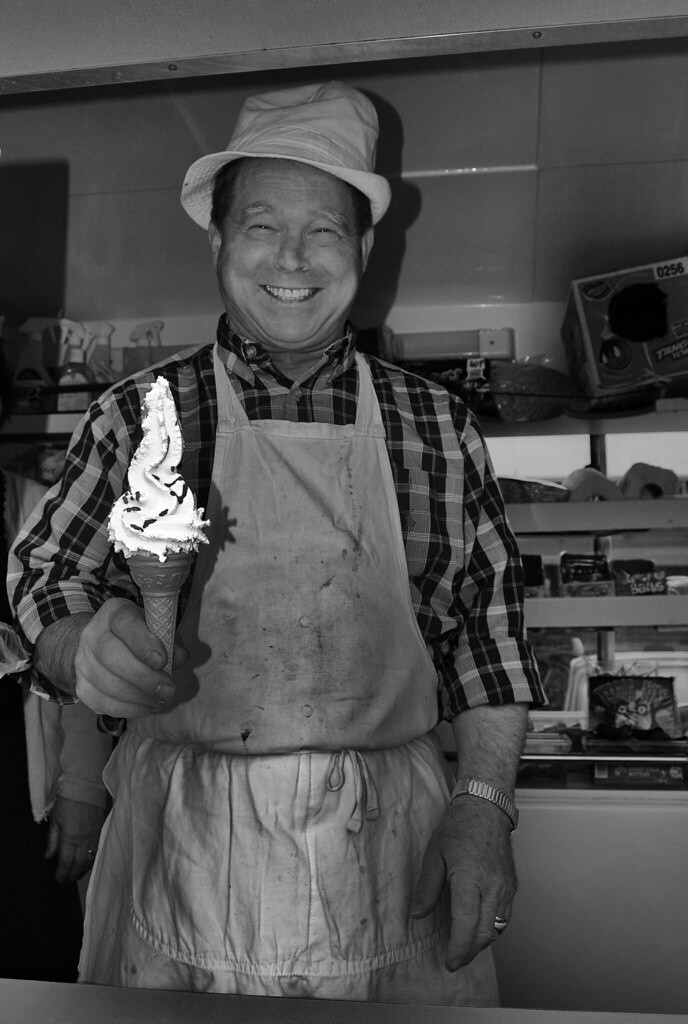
[495,915,508,931]
[89,849,96,856]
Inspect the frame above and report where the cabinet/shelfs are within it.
[472,408,688,1012]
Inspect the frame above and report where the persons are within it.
[5,78,538,1007]
[0,457,113,982]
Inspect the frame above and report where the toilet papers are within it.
[622,463,677,500]
[563,467,620,501]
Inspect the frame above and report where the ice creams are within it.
[105,375,211,676]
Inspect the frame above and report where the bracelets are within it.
[450,776,520,834]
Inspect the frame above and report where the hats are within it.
[181,80,392,232]
